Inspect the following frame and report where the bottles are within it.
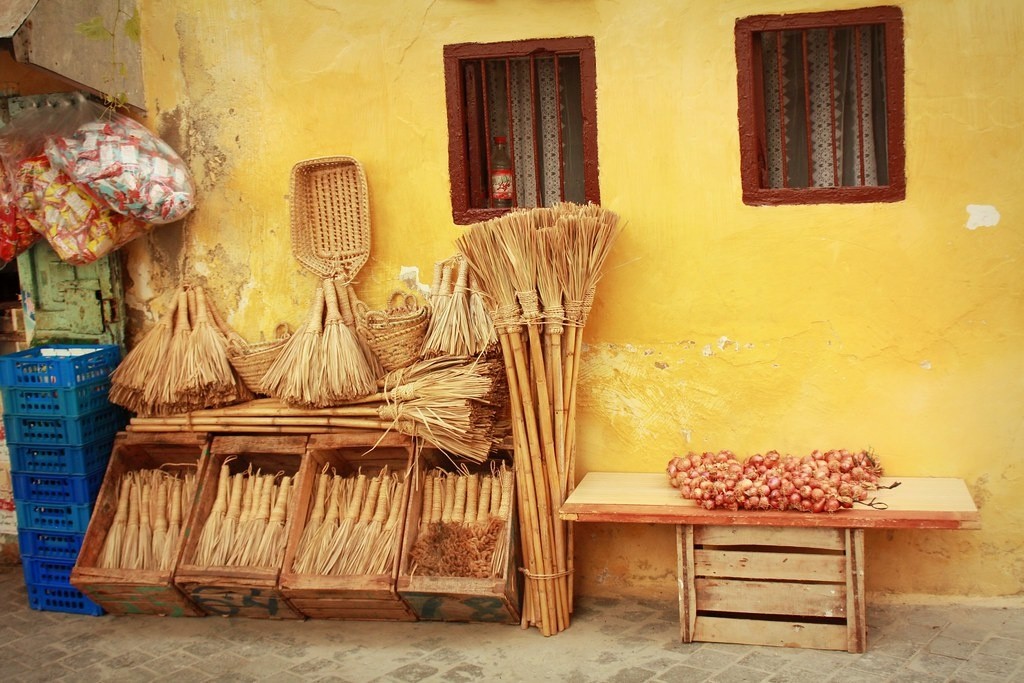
[490,136,514,206]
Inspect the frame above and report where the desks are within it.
[558,469,983,654]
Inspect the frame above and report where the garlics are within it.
[669,449,882,513]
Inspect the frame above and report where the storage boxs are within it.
[173,435,307,622]
[0,344,132,618]
[69,431,214,617]
[277,430,420,624]
[398,433,524,625]
